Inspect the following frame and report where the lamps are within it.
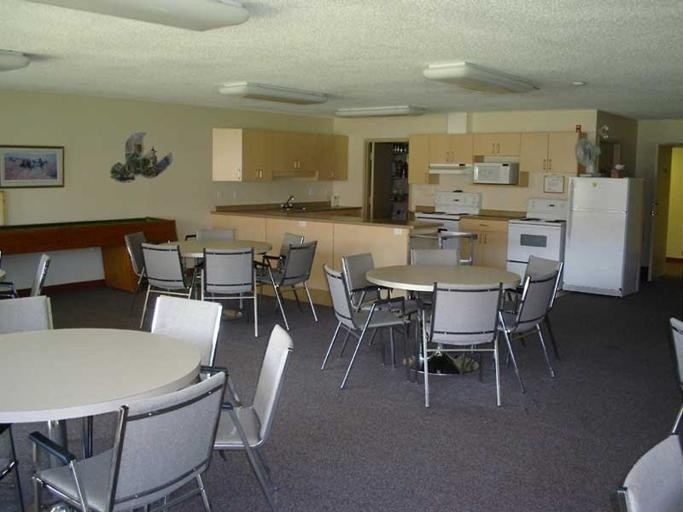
[422,60,536,97]
[1,49,31,74]
[331,106,426,120]
[217,81,332,104]
[15,1,252,33]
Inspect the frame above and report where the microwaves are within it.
[472,163,519,185]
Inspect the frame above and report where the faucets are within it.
[280,196,295,208]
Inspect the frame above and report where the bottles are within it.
[391,143,408,199]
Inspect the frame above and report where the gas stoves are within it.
[508,215,566,227]
[412,210,474,221]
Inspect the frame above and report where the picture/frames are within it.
[542,175,565,194]
[0,142,67,191]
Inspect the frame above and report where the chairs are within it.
[1,253,54,336]
[124,229,318,338]
[664,316,682,435]
[614,432,682,510]
[321,250,562,406]
[211,319,297,511]
[27,372,227,512]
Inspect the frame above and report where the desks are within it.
[0,327,204,510]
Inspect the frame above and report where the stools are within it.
[0,424,27,510]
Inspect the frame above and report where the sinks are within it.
[264,207,308,211]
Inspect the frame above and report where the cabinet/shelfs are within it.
[316,133,350,181]
[469,132,523,159]
[209,127,272,185]
[269,130,317,180]
[407,133,439,186]
[519,131,581,176]
[427,132,473,165]
[457,216,507,270]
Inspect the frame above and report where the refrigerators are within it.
[561,175,643,300]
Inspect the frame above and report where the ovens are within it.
[506,222,562,265]
[416,221,458,250]
